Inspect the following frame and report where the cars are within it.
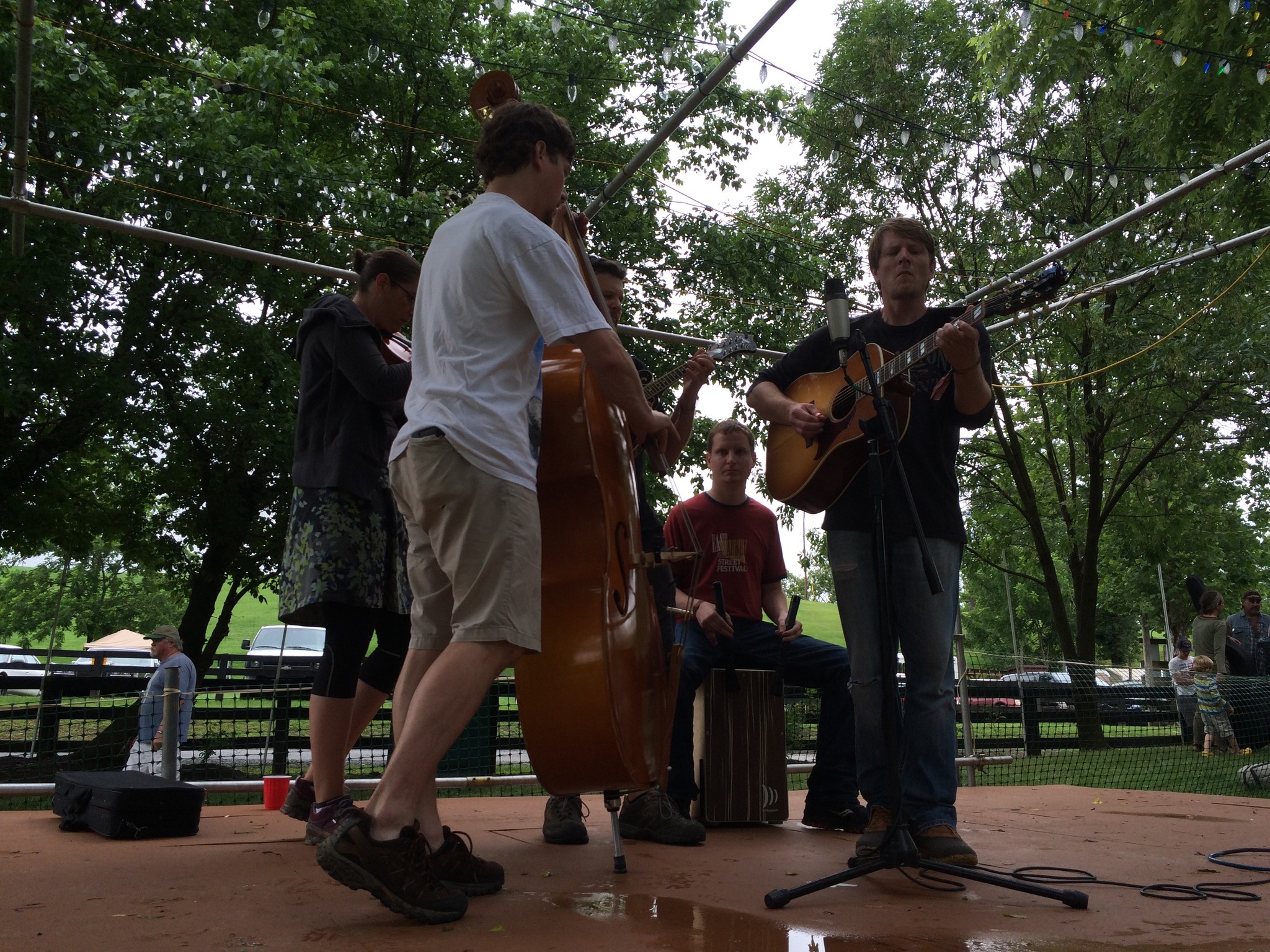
[955,678,1022,707]
[70,647,161,678]
[0,644,50,698]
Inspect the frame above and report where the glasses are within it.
[390,278,415,307]
[1244,597,1262,601]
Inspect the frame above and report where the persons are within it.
[122,626,196,781]
[275,245,421,846]
[321,104,669,924]
[662,419,868,832]
[750,217,999,868]
[543,257,716,846]
[1168,590,1270,756]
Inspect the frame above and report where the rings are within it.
[814,413,822,418]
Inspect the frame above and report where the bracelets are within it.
[157,731,162,734]
[930,355,982,400]
[693,599,704,617]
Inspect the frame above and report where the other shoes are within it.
[855,804,894,857]
[801,801,869,834]
[916,825,978,865]
[1202,751,1214,757]
[1238,748,1252,756]
[669,796,692,821]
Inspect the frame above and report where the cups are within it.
[263,776,292,810]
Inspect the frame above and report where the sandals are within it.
[1192,744,1204,752]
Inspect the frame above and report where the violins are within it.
[381,331,412,367]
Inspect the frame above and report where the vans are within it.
[895,652,960,687]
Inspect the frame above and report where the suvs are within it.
[1001,670,1110,708]
[241,624,327,702]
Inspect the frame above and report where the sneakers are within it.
[279,775,316,822]
[304,793,364,846]
[619,790,706,845]
[542,795,590,845]
[427,824,505,896]
[316,808,469,924]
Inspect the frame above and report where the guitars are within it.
[762,258,1073,514]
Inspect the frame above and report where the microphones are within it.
[825,279,850,343]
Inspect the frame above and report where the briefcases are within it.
[52,770,207,840]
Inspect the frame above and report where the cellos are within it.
[465,62,687,877]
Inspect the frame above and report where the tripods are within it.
[765,316,1091,910]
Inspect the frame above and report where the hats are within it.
[143,625,180,640]
[1176,638,1193,652]
